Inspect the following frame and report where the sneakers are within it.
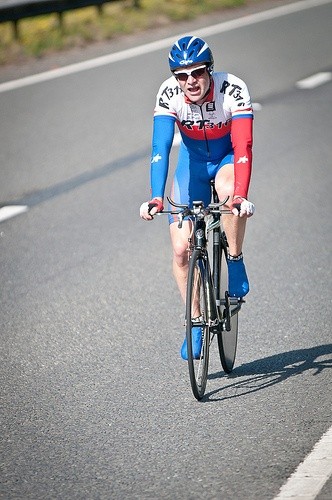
[181,326,205,361]
[226,259,250,299]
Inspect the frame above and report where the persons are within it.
[139,36,255,360]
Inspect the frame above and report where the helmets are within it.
[167,36,214,74]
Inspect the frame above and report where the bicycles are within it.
[150,196,245,399]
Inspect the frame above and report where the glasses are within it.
[174,68,207,81]
